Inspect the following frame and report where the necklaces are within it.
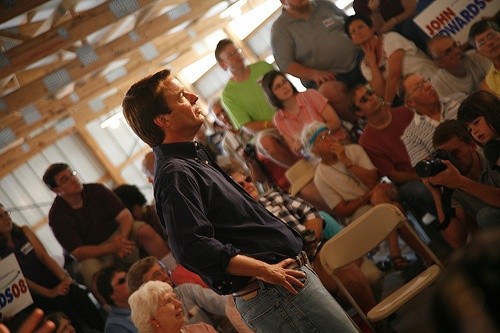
[322,158,360,186]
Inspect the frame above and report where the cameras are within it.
[415,150,460,188]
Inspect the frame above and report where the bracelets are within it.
[347,160,354,168]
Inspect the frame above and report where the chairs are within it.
[319,203,446,333]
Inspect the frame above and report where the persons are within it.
[209,95,258,174]
[457,90,500,167]
[426,33,492,97]
[122,69,360,333]
[43,163,176,317]
[399,73,468,168]
[420,118,500,254]
[215,38,299,168]
[469,19,500,99]
[343,13,438,106]
[262,70,341,158]
[301,120,410,270]
[215,155,377,333]
[0,151,251,333]
[196,122,227,160]
[271,0,368,92]
[353,0,435,60]
[346,82,444,217]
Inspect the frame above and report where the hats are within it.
[285,159,316,197]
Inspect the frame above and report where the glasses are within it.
[56,170,77,188]
[445,41,458,54]
[356,89,372,109]
[477,32,498,48]
[312,130,333,148]
[113,278,125,291]
[407,78,427,98]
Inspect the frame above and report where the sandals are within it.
[391,255,409,270]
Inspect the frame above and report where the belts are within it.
[233,255,306,297]
